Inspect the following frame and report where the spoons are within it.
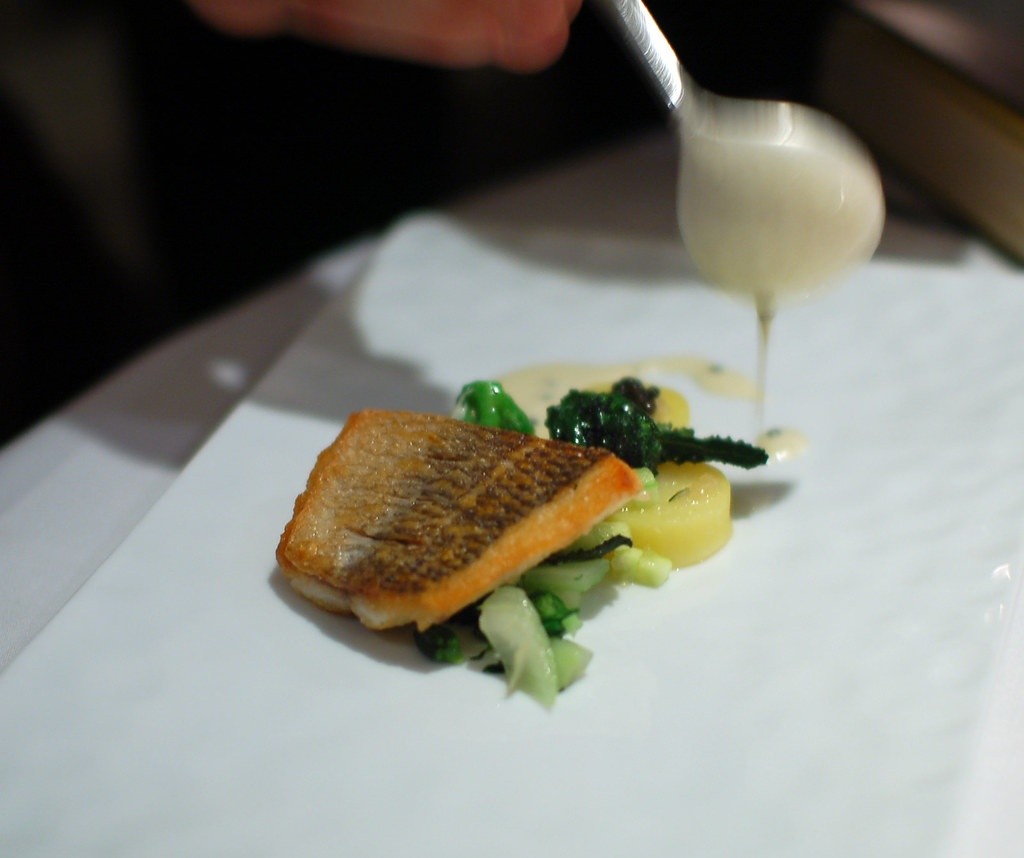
[607,1,885,298]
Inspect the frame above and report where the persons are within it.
[188,1,845,256]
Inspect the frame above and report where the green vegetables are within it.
[411,384,767,660]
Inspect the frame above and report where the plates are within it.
[0,212,1023,857]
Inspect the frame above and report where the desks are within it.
[0,129,1024,858]
